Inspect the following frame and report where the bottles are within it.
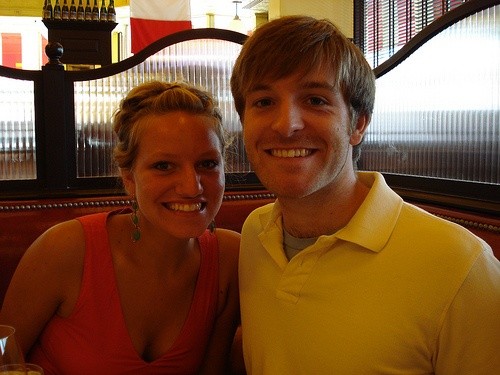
[92,0,100,23]
[85,0,92,23]
[108,0,116,23]
[100,0,107,23]
[77,0,84,23]
[70,0,76,22]
[43,0,53,21]
[53,0,62,22]
[62,0,69,23]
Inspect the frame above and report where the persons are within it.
[0,80,246,375]
[231,15,500,375]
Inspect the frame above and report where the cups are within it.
[1,363,44,375]
[0,325,16,363]
